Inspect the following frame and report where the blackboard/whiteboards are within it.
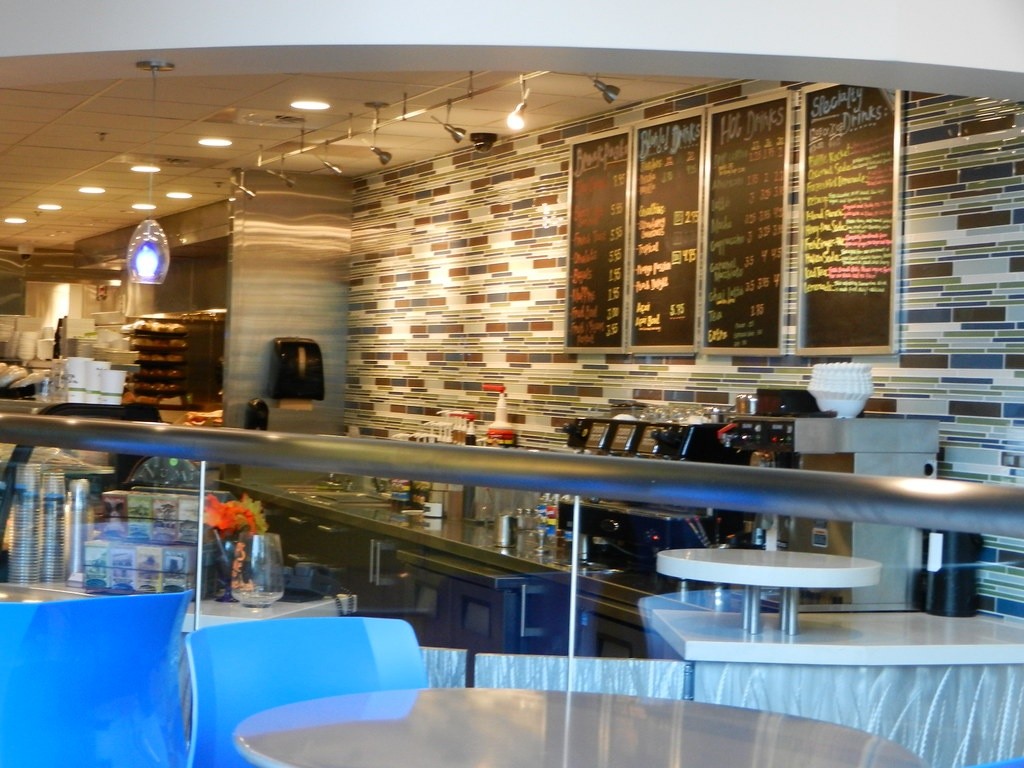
[795,82,902,357]
[628,103,707,357]
[700,87,796,357]
[563,123,628,356]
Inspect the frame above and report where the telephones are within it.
[276,561,345,603]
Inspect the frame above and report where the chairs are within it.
[0,588,197,768]
[185,615,425,768]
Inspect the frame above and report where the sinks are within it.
[275,483,362,495]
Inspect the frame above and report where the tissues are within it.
[267,337,325,411]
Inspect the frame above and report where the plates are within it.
[92,311,126,325]
[71,337,140,365]
[0,363,28,387]
[0,314,55,361]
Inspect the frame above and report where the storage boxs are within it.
[102,490,134,538]
[109,542,136,590]
[162,546,197,596]
[134,545,162,593]
[153,493,180,542]
[126,491,153,543]
[177,494,200,543]
[84,540,111,587]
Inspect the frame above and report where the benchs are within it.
[396,546,594,657]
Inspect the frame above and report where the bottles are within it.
[516,507,538,530]
[53,318,63,358]
[41,374,53,402]
[54,362,65,397]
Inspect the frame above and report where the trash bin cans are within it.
[395,548,567,654]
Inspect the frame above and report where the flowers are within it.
[203,492,270,543]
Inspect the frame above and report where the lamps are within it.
[231,175,258,199]
[314,146,343,176]
[361,132,392,165]
[127,60,174,284]
[506,81,531,130]
[264,159,296,187]
[589,77,620,105]
[429,105,466,143]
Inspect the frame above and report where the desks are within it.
[231,689,924,768]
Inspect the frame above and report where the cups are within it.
[7,463,41,583]
[40,469,67,582]
[230,533,284,608]
[644,402,703,424]
[65,479,90,577]
[66,357,127,405]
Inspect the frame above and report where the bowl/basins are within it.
[807,363,875,419]
